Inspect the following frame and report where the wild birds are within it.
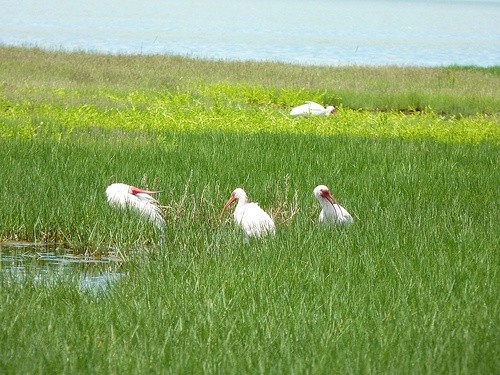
[217,187,276,245]
[313,184,355,225]
[105,182,169,234]
[289,100,337,119]
